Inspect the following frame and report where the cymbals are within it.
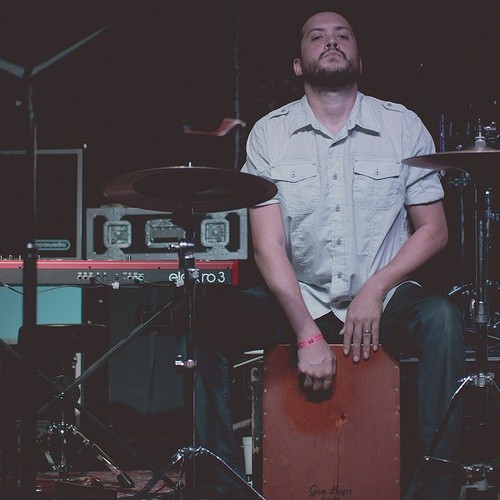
[103,167,278,214]
[402,145,500,180]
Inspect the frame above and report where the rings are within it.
[363,329,371,333]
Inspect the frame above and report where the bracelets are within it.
[297,333,325,349]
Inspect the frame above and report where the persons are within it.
[193,7,465,500]
